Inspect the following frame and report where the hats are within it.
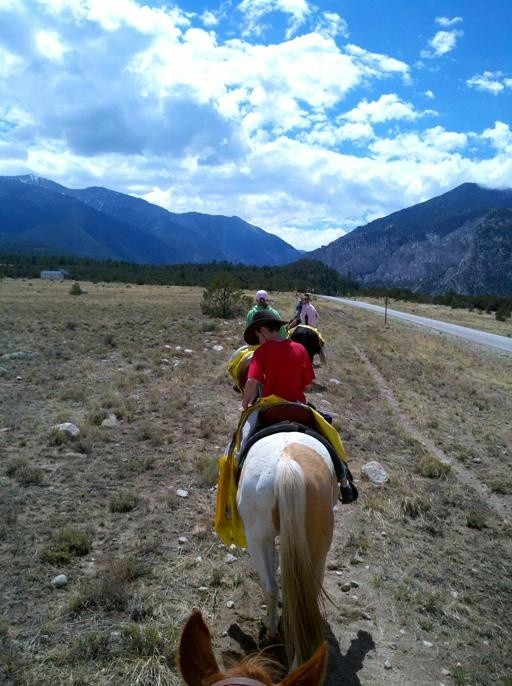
[256,290,269,302]
[243,310,290,346]
[300,292,312,301]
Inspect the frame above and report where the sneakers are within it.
[341,485,353,505]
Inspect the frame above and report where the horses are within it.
[230,419,341,663]
[174,608,331,686]
[235,349,265,401]
[290,325,321,363]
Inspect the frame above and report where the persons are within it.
[225,311,353,504]
[285,290,304,331]
[230,289,288,392]
[300,292,319,327]
